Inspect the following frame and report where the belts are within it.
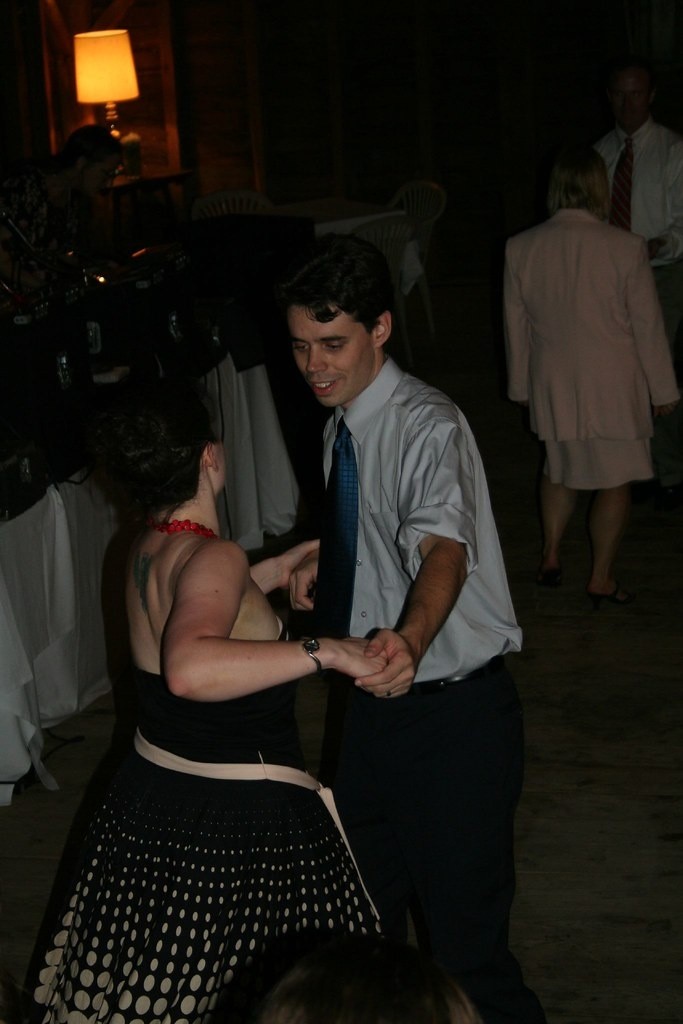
[408,656,505,695]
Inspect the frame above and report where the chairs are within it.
[191,191,272,222]
[387,179,447,335]
[101,168,203,248]
[351,215,426,362]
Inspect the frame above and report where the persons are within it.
[592,59,683,510]
[261,933,483,1024]
[504,142,681,607]
[0,124,123,288]
[281,257,543,1024]
[29,384,387,1024]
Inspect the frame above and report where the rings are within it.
[387,690,391,696]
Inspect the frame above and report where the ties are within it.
[608,137,634,232]
[313,416,358,639]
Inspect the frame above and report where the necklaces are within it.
[145,515,217,538]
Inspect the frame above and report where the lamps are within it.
[74,28,140,177]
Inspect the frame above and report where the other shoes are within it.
[630,479,659,503]
[653,483,683,509]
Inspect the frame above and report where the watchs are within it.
[302,638,322,671]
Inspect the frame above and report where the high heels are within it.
[586,580,638,611]
[536,566,563,587]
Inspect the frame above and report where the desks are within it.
[194,198,405,262]
